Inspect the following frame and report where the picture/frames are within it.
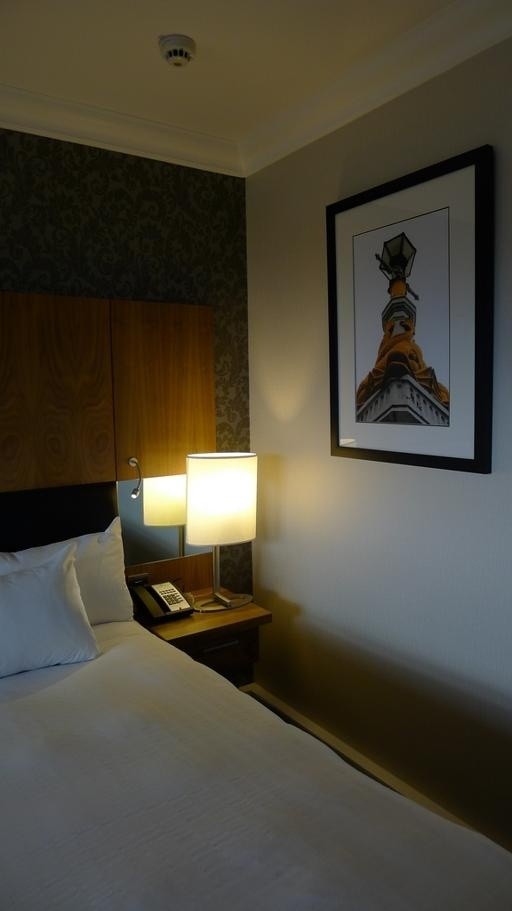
[324,143,490,473]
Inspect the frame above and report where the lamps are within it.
[143,475,186,558]
[127,457,143,498]
[184,452,257,614]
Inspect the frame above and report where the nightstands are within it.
[145,589,273,687]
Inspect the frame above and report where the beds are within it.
[2,478,511,909]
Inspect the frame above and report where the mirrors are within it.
[115,473,212,564]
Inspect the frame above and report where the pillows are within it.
[0,545,97,678]
[2,517,134,623]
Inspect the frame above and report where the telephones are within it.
[130,581,194,625]
